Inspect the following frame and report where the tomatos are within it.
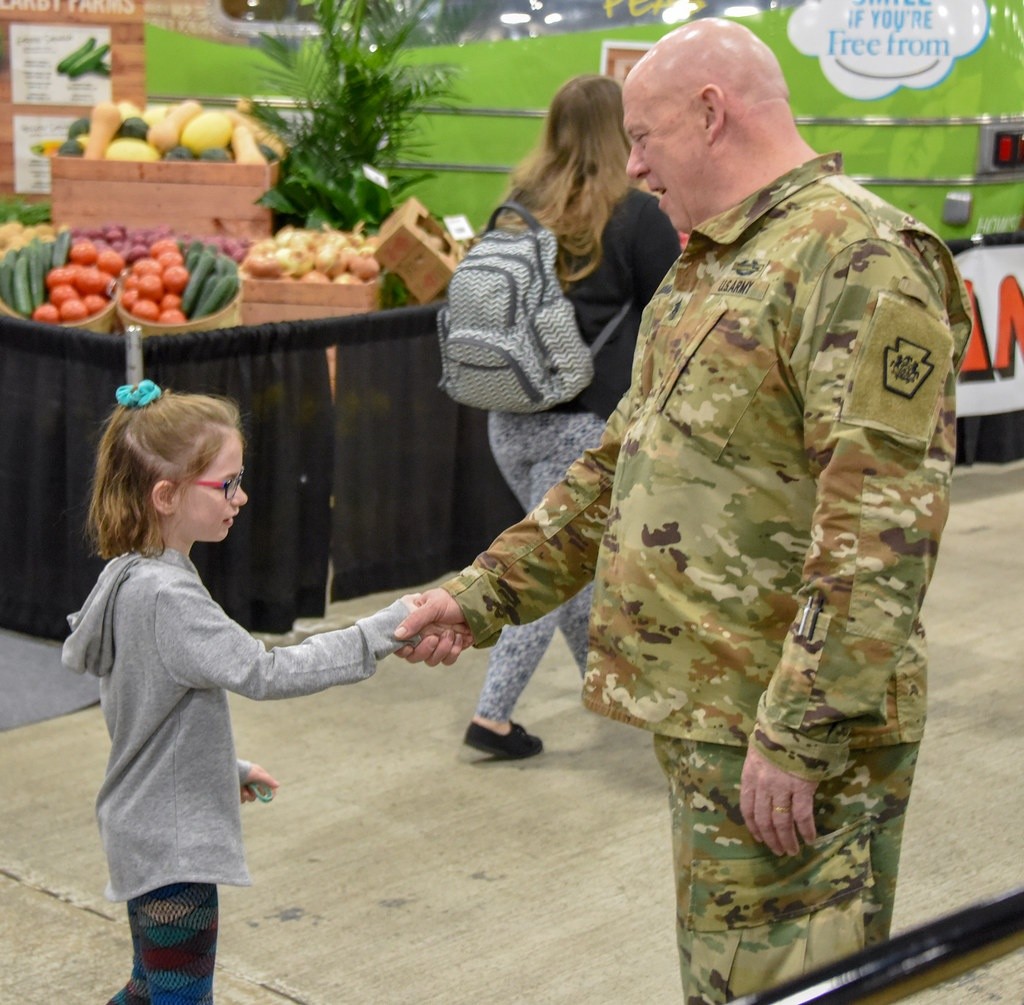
[31,237,188,327]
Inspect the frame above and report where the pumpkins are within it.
[57,95,289,167]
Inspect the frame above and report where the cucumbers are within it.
[1,232,72,319]
[56,37,113,80]
[177,239,240,323]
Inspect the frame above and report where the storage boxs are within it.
[118,248,241,339]
[374,193,465,303]
[1,242,117,334]
[50,155,281,242]
[236,227,388,327]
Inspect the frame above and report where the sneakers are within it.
[463,720,542,759]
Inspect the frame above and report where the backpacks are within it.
[436,188,631,411]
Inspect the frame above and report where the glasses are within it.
[173,468,244,497]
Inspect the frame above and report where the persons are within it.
[457,70,684,758]
[58,380,478,1004]
[384,14,974,1005]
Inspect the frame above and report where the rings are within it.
[769,804,793,815]
